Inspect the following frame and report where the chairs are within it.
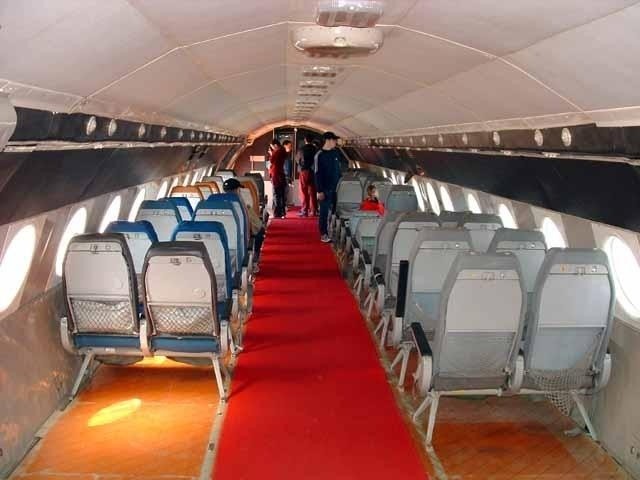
[58,169,269,398]
[324,167,614,447]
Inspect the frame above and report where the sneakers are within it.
[321,234,331,243]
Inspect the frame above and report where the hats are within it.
[322,131,340,139]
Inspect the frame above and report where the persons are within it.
[314,131,342,242]
[295,134,319,216]
[268,139,287,219]
[283,140,292,211]
[359,185,384,216]
[223,178,265,273]
